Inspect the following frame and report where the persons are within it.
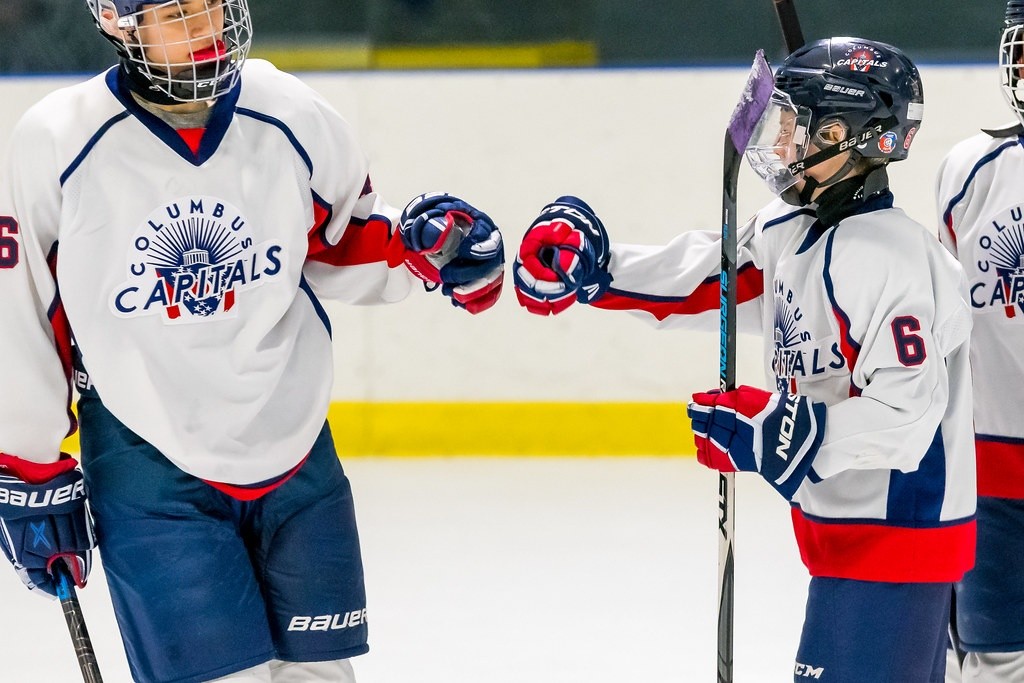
[0,0,505,683]
[919,0,1024,683]
[513,37,976,683]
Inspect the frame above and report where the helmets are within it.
[999,0,1024,127]
[85,0,253,105]
[769,37,925,164]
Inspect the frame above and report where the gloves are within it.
[513,195,614,316]
[0,451,98,601]
[687,384,827,502]
[400,191,506,315]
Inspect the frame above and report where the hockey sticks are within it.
[708,47,776,683]
[51,555,104,683]
